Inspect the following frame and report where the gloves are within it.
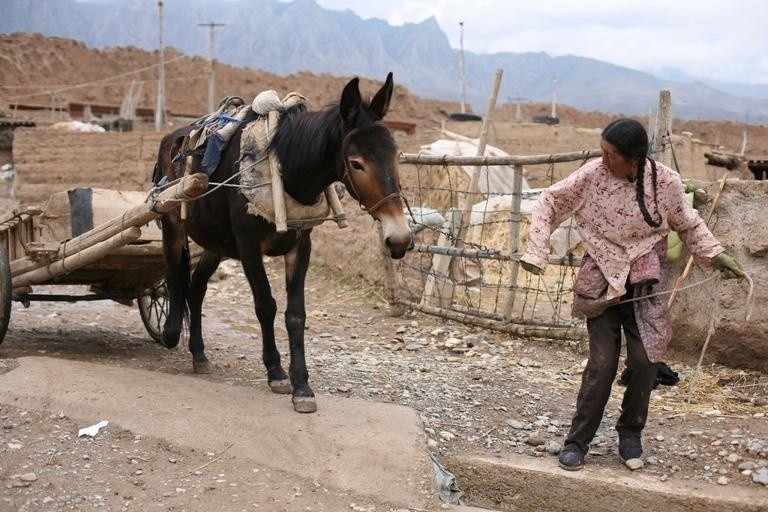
[521,262,544,276]
[710,253,744,285]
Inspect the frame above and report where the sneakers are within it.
[559,442,585,472]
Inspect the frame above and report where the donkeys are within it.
[154,72,414,412]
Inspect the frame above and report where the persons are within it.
[519,119,746,472]
